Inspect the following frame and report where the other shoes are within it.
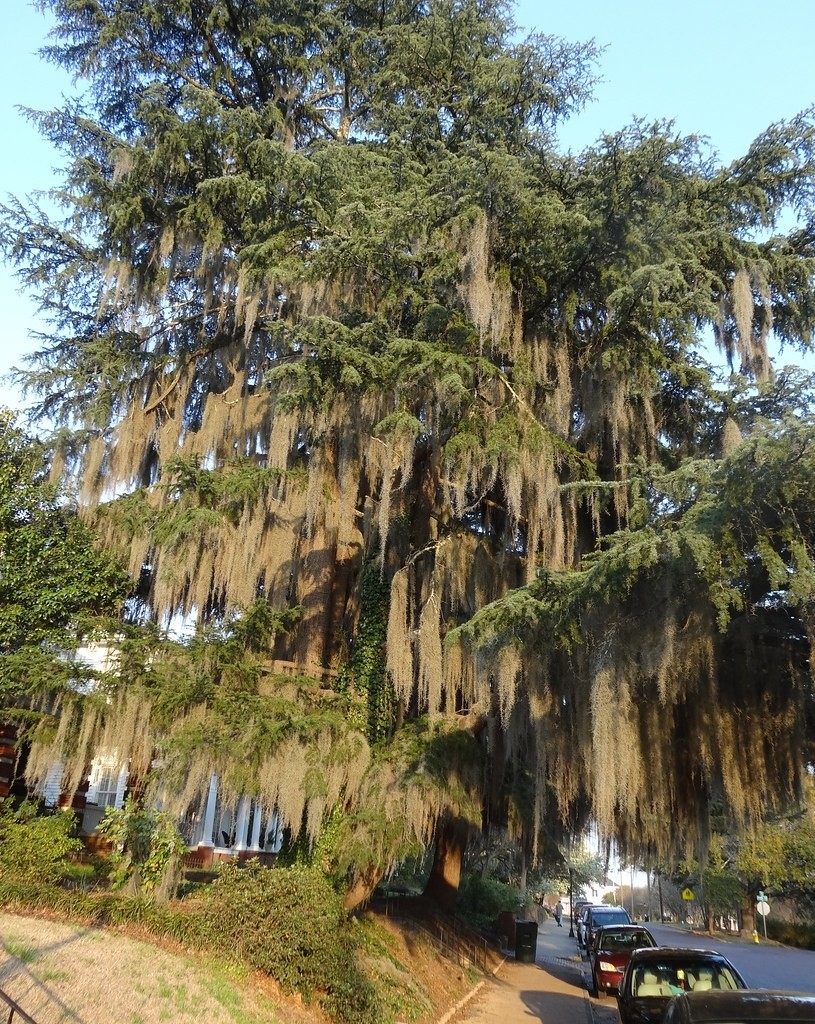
[559,924,562,927]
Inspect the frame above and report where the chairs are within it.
[638,970,672,996]
[693,968,731,991]
[602,936,619,951]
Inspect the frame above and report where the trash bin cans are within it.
[515,919,537,965]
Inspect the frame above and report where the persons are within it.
[556,900,564,927]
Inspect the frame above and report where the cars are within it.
[574,900,814,1024]
